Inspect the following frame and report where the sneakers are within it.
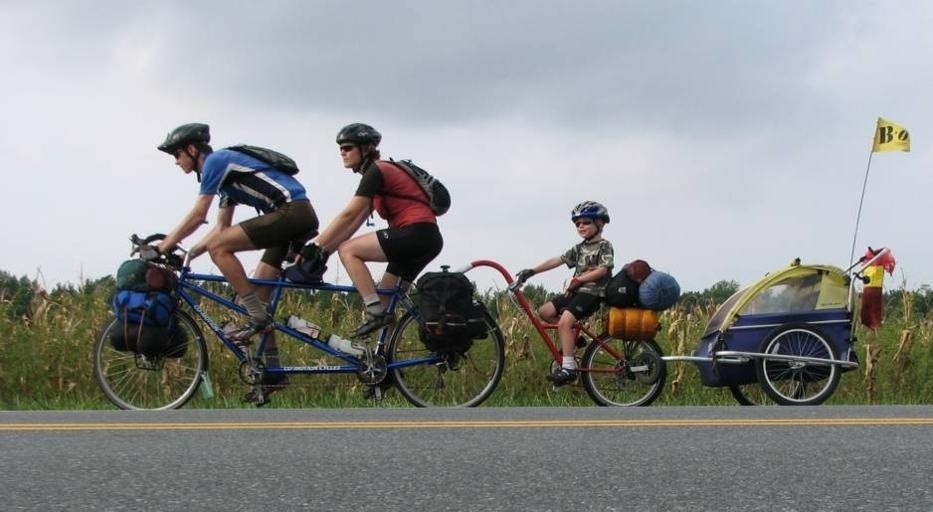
[362,368,403,399]
[349,311,394,338]
[223,314,277,341]
[546,368,576,381]
[239,373,289,403]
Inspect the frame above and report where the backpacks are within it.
[372,157,450,216]
[225,145,298,176]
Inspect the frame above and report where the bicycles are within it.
[92,232,506,411]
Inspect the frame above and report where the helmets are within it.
[156,123,210,154]
[571,201,608,223]
[335,123,381,146]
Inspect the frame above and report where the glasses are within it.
[575,221,590,227]
[172,151,182,160]
[339,145,352,151]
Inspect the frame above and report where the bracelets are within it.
[312,238,321,250]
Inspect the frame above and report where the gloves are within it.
[130,246,159,260]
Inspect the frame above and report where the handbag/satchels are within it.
[416,272,487,352]
[605,260,680,339]
[110,260,186,358]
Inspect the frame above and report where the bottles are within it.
[325,333,367,364]
[559,336,585,357]
[277,312,321,340]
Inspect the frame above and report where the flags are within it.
[871,116,911,154]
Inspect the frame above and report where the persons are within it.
[518,200,613,385]
[293,124,443,398]
[138,123,318,403]
